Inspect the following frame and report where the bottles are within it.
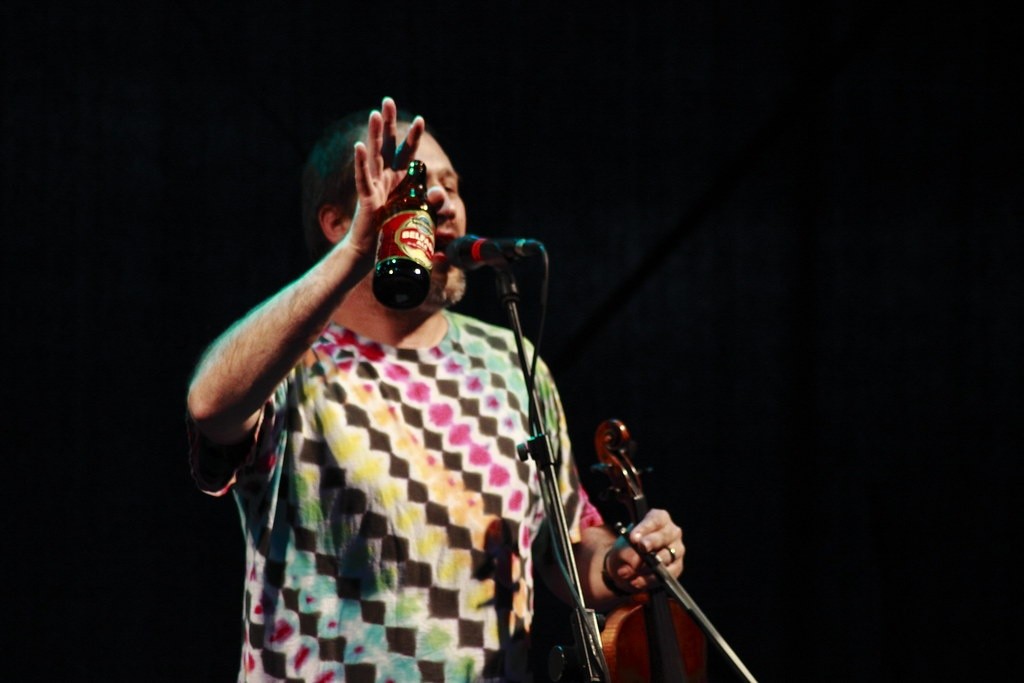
[372,159,436,311]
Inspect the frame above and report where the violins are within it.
[594,419,711,682]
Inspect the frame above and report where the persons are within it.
[187,96,685,682]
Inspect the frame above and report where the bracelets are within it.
[601,549,641,596]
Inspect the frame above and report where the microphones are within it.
[446,234,543,268]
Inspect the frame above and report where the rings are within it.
[662,544,675,562]
